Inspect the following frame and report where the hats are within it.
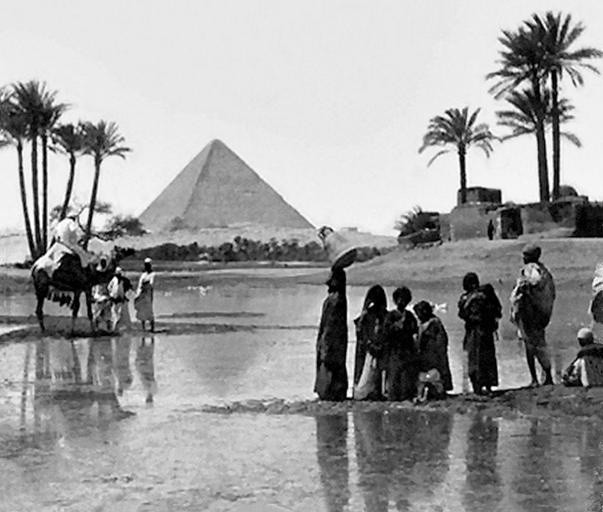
[576,327,593,339]
[522,242,541,258]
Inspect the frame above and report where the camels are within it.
[27,241,134,333]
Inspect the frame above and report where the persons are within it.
[312,270,348,400]
[55,212,94,288]
[412,300,452,401]
[457,273,502,399]
[508,243,556,390]
[317,415,603,512]
[555,261,603,388]
[90,251,157,332]
[352,285,391,401]
[380,287,418,401]
[97,336,157,404]
